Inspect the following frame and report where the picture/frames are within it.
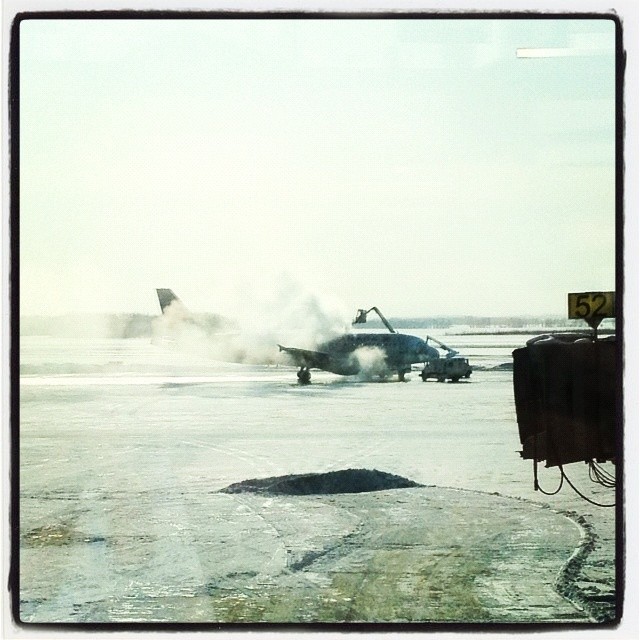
[0,14,635,629]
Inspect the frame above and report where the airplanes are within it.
[156,286,440,383]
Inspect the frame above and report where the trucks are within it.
[463,360,472,377]
[421,358,465,382]
